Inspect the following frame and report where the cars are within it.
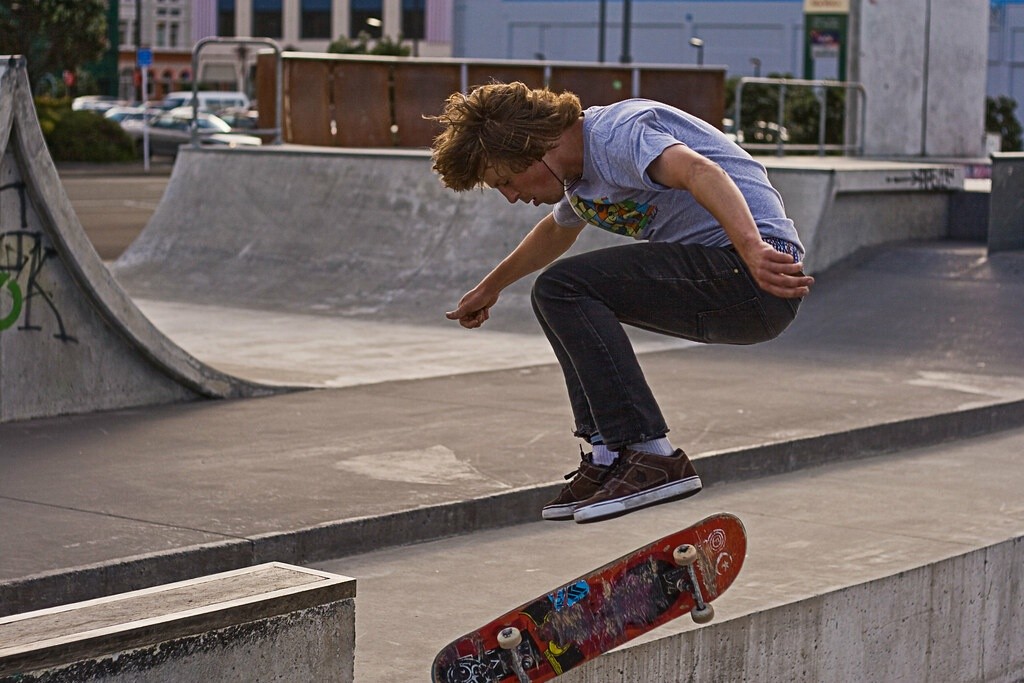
[105,90,262,159]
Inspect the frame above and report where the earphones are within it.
[529,144,546,162]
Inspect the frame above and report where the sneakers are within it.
[574,448,703,523]
[541,447,618,520]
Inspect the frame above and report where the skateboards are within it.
[430,512,749,683]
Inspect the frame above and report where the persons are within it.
[429,82,816,525]
